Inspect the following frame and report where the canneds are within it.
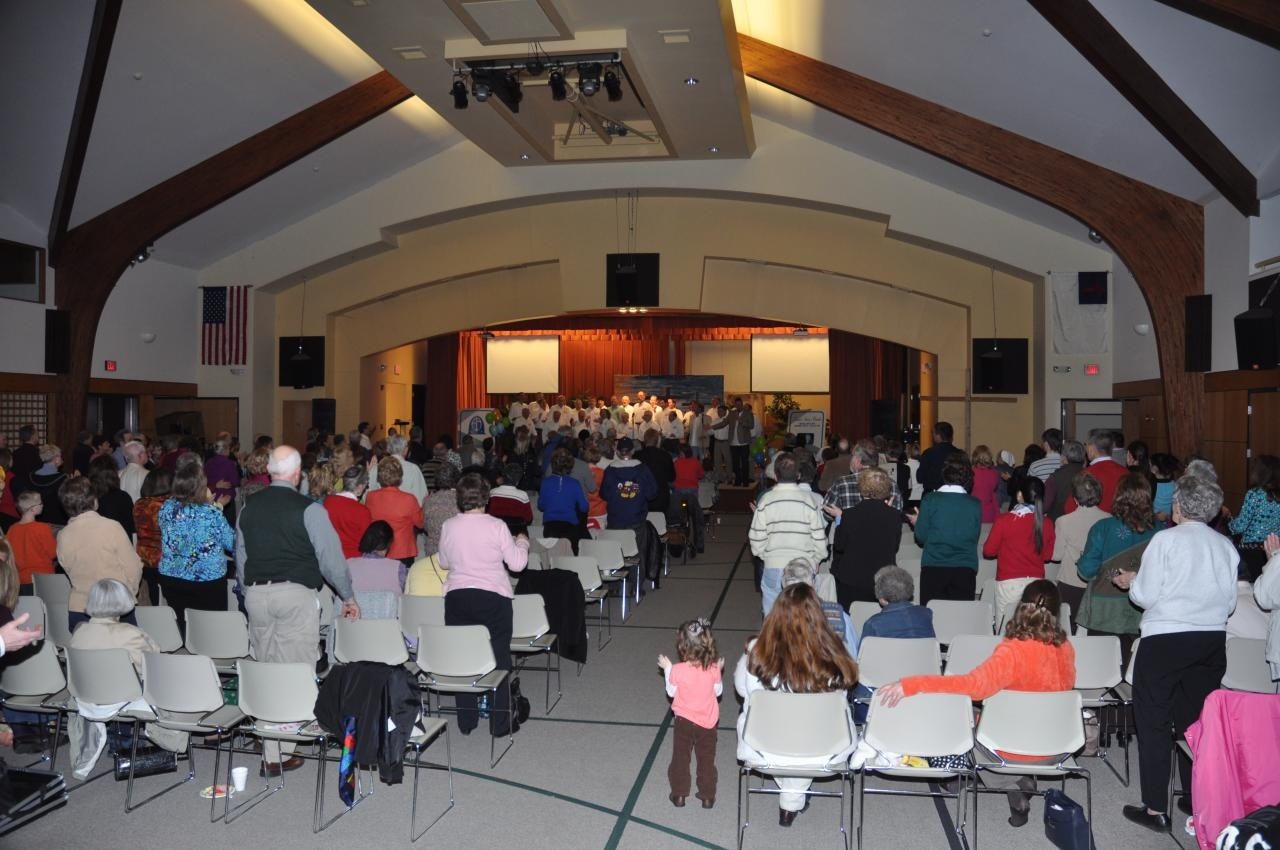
[717,517,720,524]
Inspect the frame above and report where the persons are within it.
[734,582,860,828]
[983,476,1056,634]
[0,388,1280,744]
[875,579,1077,827]
[748,452,828,621]
[831,468,905,617]
[1074,473,1169,749]
[5,490,55,596]
[657,615,725,809]
[55,475,144,636]
[438,472,530,737]
[906,463,979,670]
[236,444,361,778]
[1112,473,1240,834]
[70,576,159,781]
[156,461,235,649]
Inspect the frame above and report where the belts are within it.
[249,579,288,586]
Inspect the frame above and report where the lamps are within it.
[448,65,471,111]
[468,74,493,104]
[575,60,602,97]
[546,63,568,103]
[603,63,624,104]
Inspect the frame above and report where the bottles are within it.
[479,693,490,719]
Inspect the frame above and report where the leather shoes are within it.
[204,730,238,746]
[259,751,305,777]
[1179,797,1195,814]
[1123,805,1171,832]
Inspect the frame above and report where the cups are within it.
[232,767,248,791]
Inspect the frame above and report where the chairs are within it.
[0,493,1280,850]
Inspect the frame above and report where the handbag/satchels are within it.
[1044,786,1097,850]
[510,677,530,723]
[1076,715,1100,755]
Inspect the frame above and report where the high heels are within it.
[1099,732,1112,746]
[1117,731,1130,747]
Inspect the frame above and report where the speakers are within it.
[971,338,1028,395]
[1235,307,1279,370]
[607,252,660,307]
[278,336,325,389]
[1184,293,1212,372]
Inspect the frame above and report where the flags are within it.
[201,287,249,366]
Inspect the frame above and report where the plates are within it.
[200,784,235,799]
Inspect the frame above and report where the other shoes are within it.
[670,791,691,807]
[800,787,813,814]
[734,484,740,487]
[696,791,716,808]
[463,724,478,734]
[779,807,799,828]
[1006,775,1036,827]
[631,589,645,596]
[496,724,521,737]
[743,481,748,487]
[13,732,70,753]
